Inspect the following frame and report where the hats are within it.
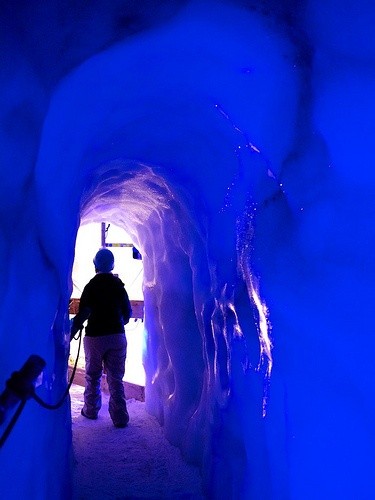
[93,249,114,272]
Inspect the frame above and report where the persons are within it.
[71,249,132,429]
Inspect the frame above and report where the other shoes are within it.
[80,408,97,419]
[114,423,127,427]
